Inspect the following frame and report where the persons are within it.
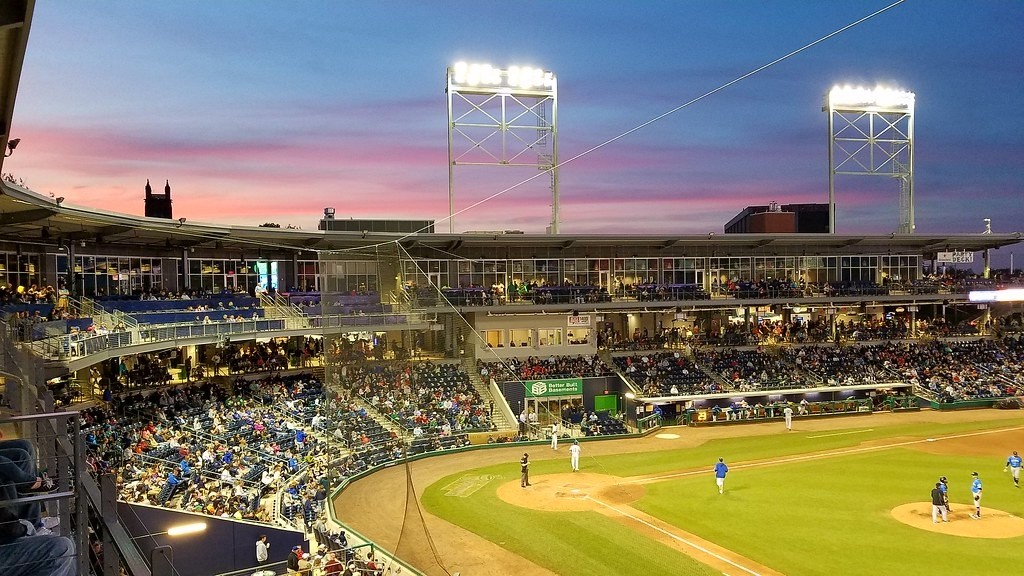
[970,472,982,519]
[784,405,793,431]
[937,477,951,515]
[931,483,950,525]
[520,453,531,488]
[569,440,581,472]
[1006,450,1024,488]
[551,422,558,450]
[0,275,1024,576]
[714,457,728,494]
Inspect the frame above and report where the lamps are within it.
[4,138,21,157]
[56,197,65,206]
[176,218,186,228]
[362,228,369,239]
[708,232,715,240]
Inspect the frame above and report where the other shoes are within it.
[971,514,981,519]
[933,521,940,523]
[942,519,950,523]
[1014,482,1019,488]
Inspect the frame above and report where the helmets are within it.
[939,476,949,483]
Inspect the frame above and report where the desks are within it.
[132,309,265,324]
[290,295,379,305]
[638,283,697,293]
[72,331,132,357]
[302,305,392,315]
[442,288,490,297]
[94,293,256,301]
[140,319,285,339]
[534,285,597,294]
[313,316,407,327]
[0,304,55,321]
[95,298,260,313]
[20,318,93,342]
[282,290,379,297]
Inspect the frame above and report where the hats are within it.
[935,483,943,488]
[1013,451,1017,455]
[970,472,978,476]
